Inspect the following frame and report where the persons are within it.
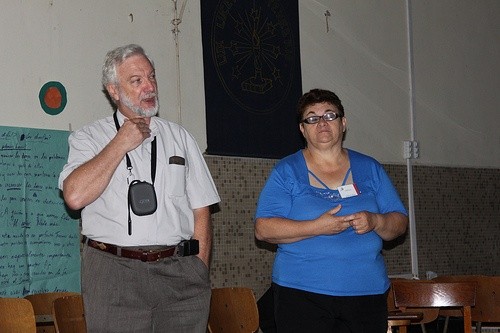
[56,44,221,333]
[253,90,409,333]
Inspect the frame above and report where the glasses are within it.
[299,113,341,124]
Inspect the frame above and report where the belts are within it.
[80,236,176,262]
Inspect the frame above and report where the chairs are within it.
[0,272,500,333]
